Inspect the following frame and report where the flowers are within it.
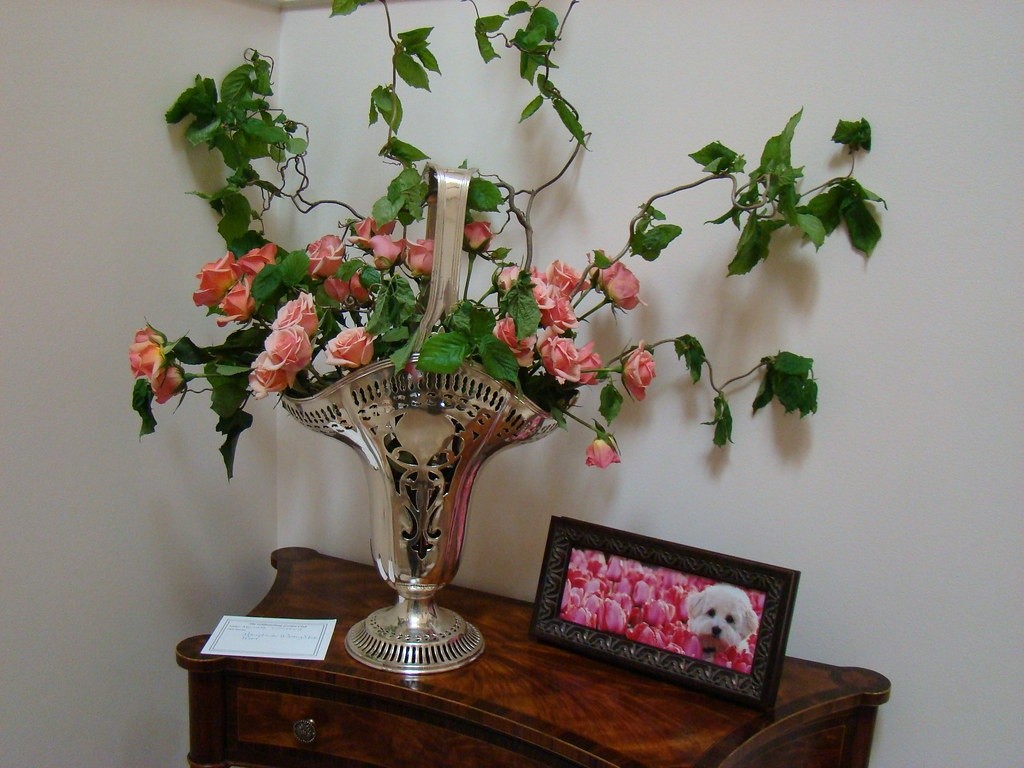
[126,0,890,469]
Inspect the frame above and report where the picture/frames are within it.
[529,514,802,711]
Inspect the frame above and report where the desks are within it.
[172,544,892,768]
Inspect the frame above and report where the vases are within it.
[279,166,558,674]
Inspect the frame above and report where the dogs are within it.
[685,583,758,662]
